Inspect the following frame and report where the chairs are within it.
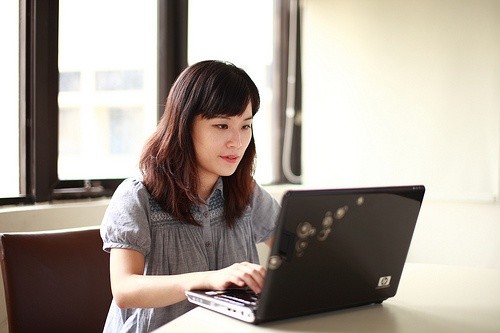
[0,225,114,333]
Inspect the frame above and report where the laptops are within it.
[184,184,426,325]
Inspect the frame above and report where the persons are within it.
[99,60,282,333]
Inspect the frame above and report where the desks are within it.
[149,199,500,333]
